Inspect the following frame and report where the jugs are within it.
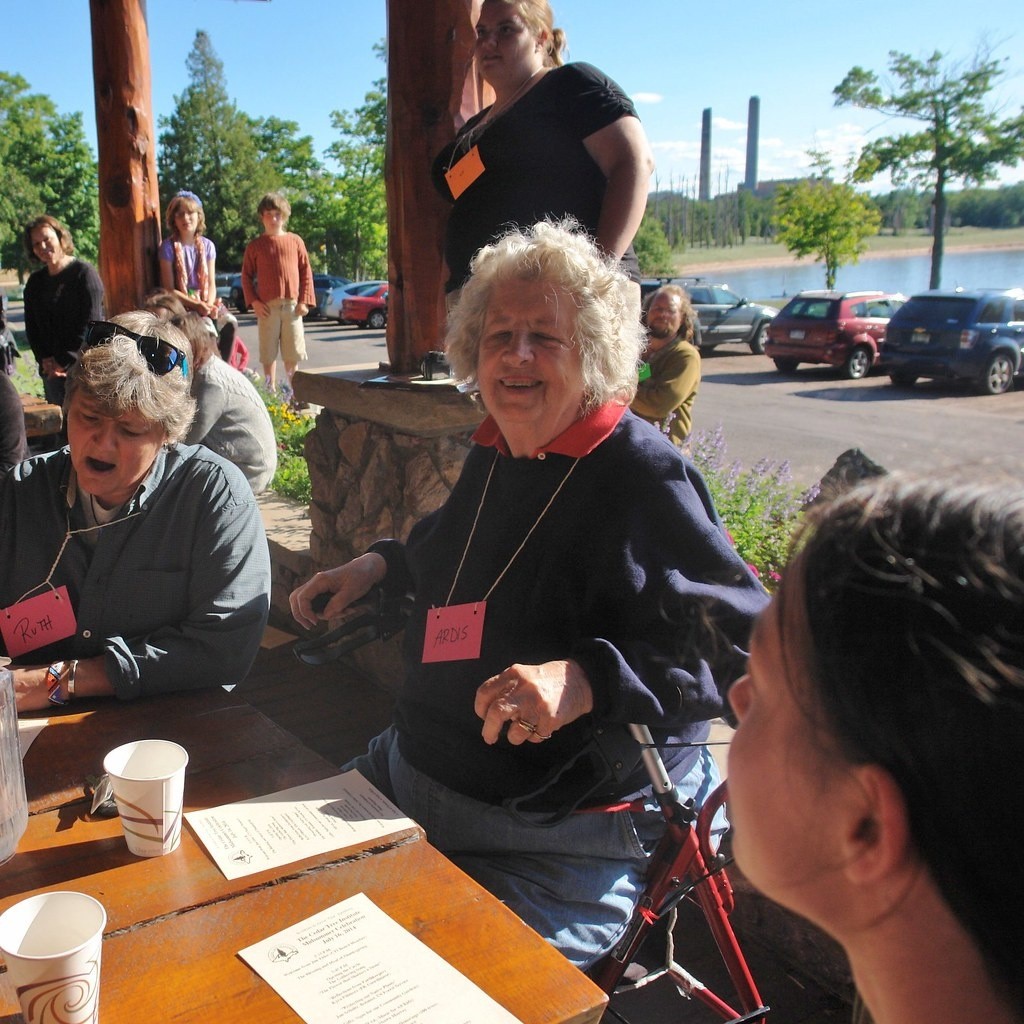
[0,657,28,867]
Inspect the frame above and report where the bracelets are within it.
[46,661,68,708]
[67,660,78,704]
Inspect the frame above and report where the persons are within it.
[630,284,703,442]
[431,0,649,329]
[0,295,30,479]
[242,193,317,397]
[158,190,218,321]
[25,214,105,406]
[140,291,278,495]
[289,219,776,971]
[0,311,272,714]
[724,472,1023,1023]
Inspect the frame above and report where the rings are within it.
[532,732,552,740]
[517,720,536,733]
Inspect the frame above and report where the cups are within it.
[0,891,107,1024]
[104,739,189,857]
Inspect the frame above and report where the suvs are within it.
[640,274,780,356]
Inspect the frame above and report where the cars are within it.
[880,288,1024,395]
[216,273,389,328]
[761,291,911,380]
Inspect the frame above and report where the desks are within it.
[0,687,611,1023]
[17,391,63,438]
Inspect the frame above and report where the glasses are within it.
[86,319,187,381]
